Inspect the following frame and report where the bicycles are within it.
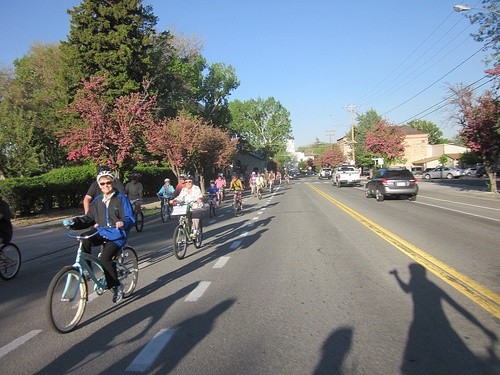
[216,188,226,205]
[206,192,217,218]
[158,193,174,222]
[129,198,144,232]
[171,201,204,260]
[46,218,139,334]
[0,238,22,280]
[231,189,244,215]
[248,175,282,201]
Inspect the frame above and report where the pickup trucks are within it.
[331,165,360,188]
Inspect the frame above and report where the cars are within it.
[363,167,418,201]
[361,167,369,175]
[423,165,462,180]
[462,166,500,178]
[287,167,314,180]
[410,167,425,175]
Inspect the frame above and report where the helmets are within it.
[164,173,193,182]
[96,170,115,186]
[130,172,138,180]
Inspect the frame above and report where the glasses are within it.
[100,181,113,185]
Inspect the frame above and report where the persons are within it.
[123,174,141,215]
[0,195,14,244]
[229,175,243,211]
[206,180,220,212]
[81,166,127,215]
[168,175,205,241]
[214,175,227,198]
[156,177,176,214]
[172,173,187,198]
[61,170,136,303]
[248,171,290,197]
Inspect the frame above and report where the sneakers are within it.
[112,283,126,302]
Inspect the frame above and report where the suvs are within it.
[318,167,333,179]
